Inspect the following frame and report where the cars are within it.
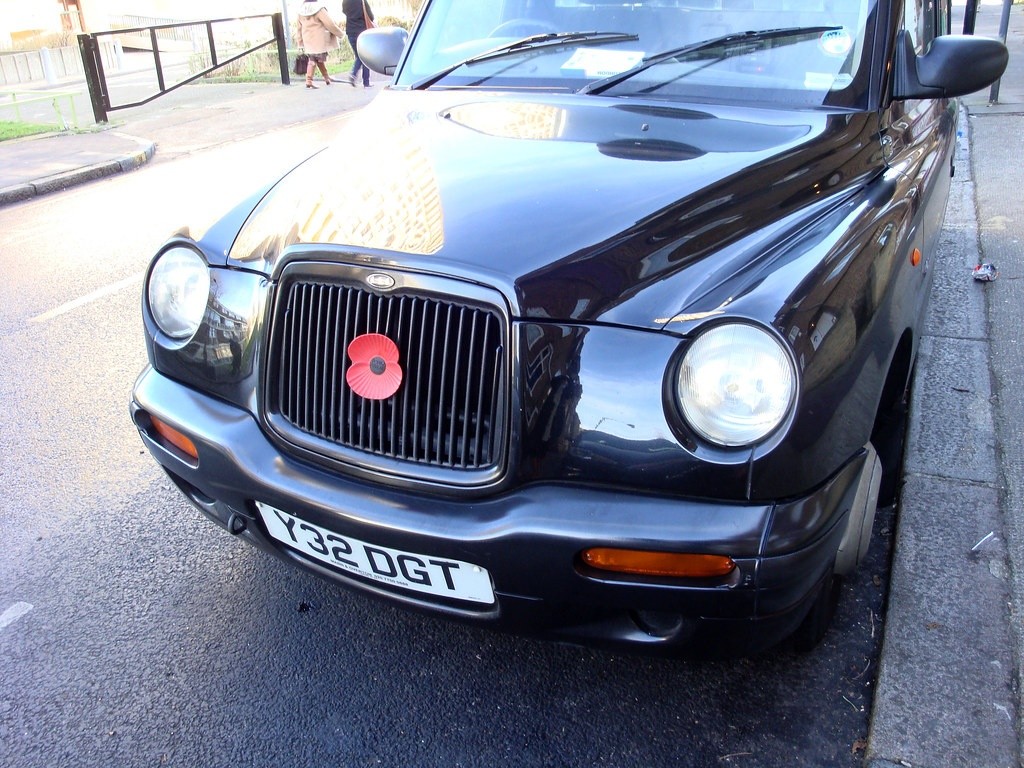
[130,0,1009,659]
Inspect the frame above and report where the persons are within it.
[297,0,344,89]
[342,0,374,88]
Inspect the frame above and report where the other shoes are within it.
[326,79,333,85]
[307,84,319,89]
[364,84,375,88]
[350,75,357,88]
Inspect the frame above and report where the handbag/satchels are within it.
[362,0,377,29]
[294,48,309,75]
